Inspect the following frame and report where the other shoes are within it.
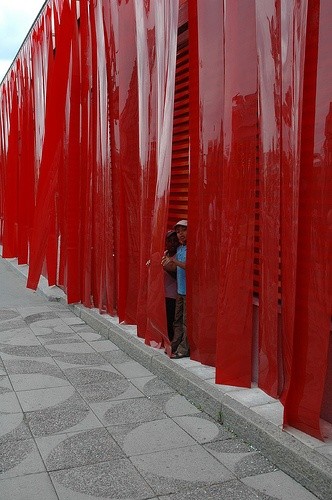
[171,351,187,359]
[164,347,176,354]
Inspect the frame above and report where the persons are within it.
[162,219,189,360]
[145,229,180,355]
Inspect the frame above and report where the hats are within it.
[173,219,187,226]
[166,230,175,237]
